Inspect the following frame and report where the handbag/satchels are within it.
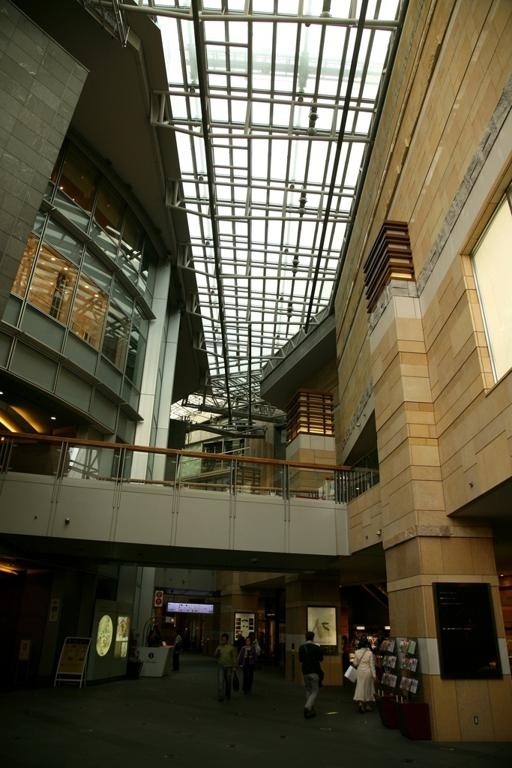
[233,672,239,690]
[344,665,357,683]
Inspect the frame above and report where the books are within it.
[380,638,418,695]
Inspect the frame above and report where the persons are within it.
[171,630,182,674]
[298,631,324,719]
[148,625,164,648]
[341,635,350,674]
[250,632,261,656]
[214,633,237,703]
[352,639,378,714]
[239,636,256,696]
